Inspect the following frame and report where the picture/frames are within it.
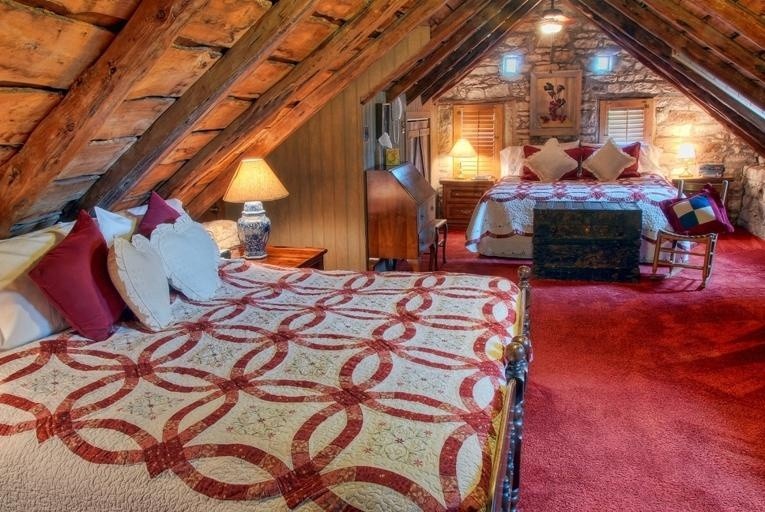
[528,69,583,136]
[377,101,392,141]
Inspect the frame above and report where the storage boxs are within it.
[532,201,643,283]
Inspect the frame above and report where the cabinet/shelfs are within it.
[367,161,436,258]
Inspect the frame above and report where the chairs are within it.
[652,179,729,287]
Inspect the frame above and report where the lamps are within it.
[593,50,613,76]
[501,52,521,77]
[539,0,568,35]
[677,142,696,178]
[223,155,290,260]
[447,137,478,180]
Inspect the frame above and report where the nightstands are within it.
[670,177,734,221]
[439,176,496,232]
[220,243,329,272]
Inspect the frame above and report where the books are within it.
[698,162,725,178]
[472,175,492,180]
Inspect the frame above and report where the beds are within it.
[0,260,531,512]
[476,174,693,264]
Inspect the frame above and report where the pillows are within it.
[498,136,660,185]
[658,183,734,235]
[0,188,222,356]
